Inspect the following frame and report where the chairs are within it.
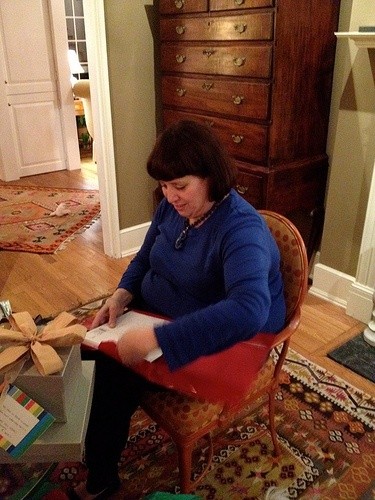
[140,209,309,493]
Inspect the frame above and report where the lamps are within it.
[69,50,85,81]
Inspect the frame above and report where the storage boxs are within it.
[0,311,96,465]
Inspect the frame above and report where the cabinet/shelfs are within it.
[153,0,342,265]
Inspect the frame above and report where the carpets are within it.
[0,289,375,500]
[0,185,101,255]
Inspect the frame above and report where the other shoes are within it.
[68,475,120,500]
